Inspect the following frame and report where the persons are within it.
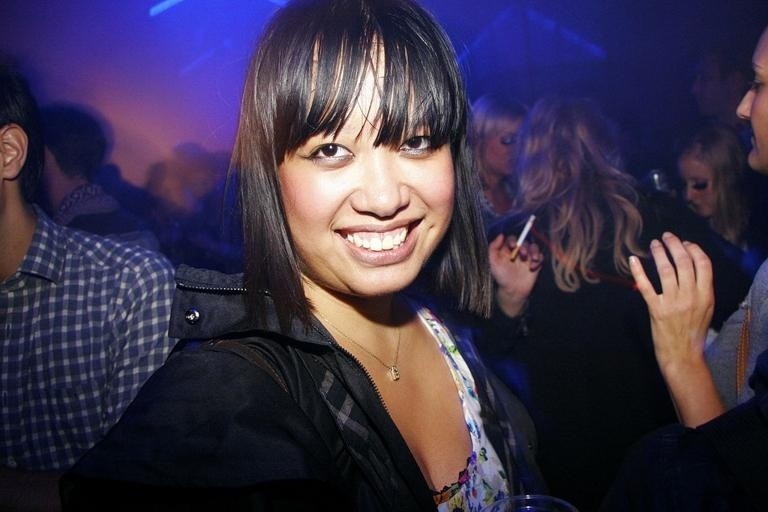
[58,2,540,511]
[469,27,768,511]
[2,69,248,510]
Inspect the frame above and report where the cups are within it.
[478,490,577,512]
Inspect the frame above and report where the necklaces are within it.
[313,310,401,381]
